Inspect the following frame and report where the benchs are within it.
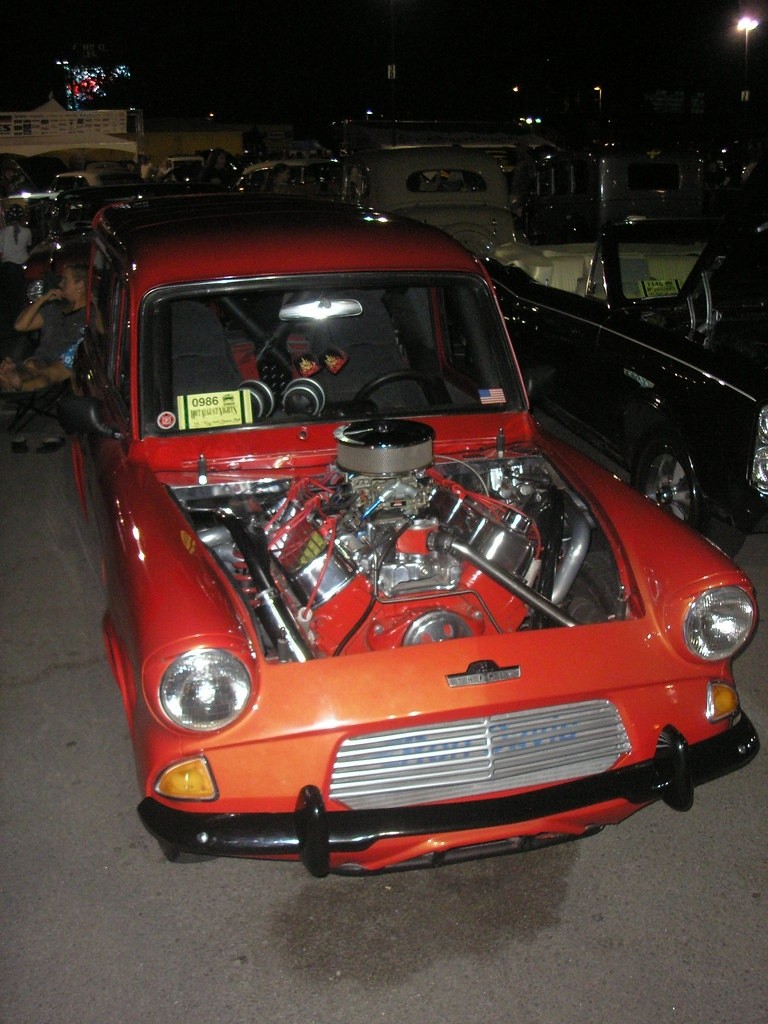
[549,254,698,299]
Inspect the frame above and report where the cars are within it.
[53,191,764,879]
[254,109,768,558]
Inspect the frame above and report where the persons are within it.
[204,147,234,195]
[0,205,34,321]
[126,161,137,174]
[270,162,291,194]
[0,261,104,394]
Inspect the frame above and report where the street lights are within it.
[733,12,760,120]
[594,86,602,113]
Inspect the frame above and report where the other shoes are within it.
[35,435,65,454]
[12,437,29,454]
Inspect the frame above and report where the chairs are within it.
[151,302,244,415]
[316,289,428,413]
[0,271,112,435]
